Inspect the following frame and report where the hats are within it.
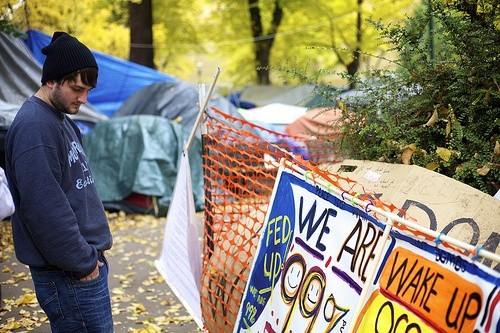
[40,30,99,85]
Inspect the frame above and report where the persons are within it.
[2,31,115,333]
